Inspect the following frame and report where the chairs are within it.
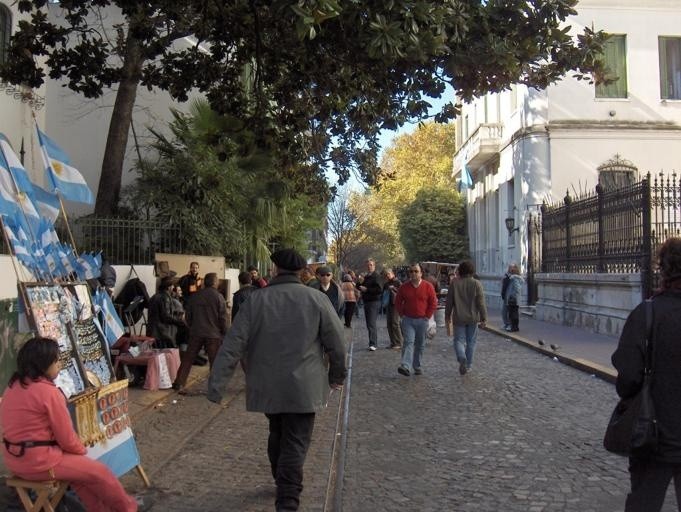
[125,309,156,348]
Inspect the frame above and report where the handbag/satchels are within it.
[604,298,662,462]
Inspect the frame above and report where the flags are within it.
[1,132,61,240]
[92,288,126,348]
[0,217,104,283]
[31,112,99,205]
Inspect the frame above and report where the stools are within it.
[7,476,67,512]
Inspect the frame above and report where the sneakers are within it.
[413,367,423,376]
[459,357,468,376]
[398,366,411,376]
[367,345,376,351]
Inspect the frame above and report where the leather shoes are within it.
[385,344,401,350]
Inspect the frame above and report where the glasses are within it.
[320,273,331,277]
[408,269,421,273]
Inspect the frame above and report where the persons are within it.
[232,271,257,324]
[0,336,139,512]
[497,263,515,331]
[609,238,681,512]
[300,267,317,287]
[146,275,189,348]
[171,284,184,319]
[338,270,356,328]
[264,267,276,282]
[394,263,438,376]
[380,267,405,349]
[172,273,226,391]
[355,258,385,351]
[179,262,202,297]
[444,261,487,375]
[501,262,525,332]
[247,265,268,289]
[348,268,361,321]
[309,265,345,321]
[207,248,348,511]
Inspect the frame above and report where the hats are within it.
[157,276,183,291]
[316,266,332,274]
[269,247,308,272]
